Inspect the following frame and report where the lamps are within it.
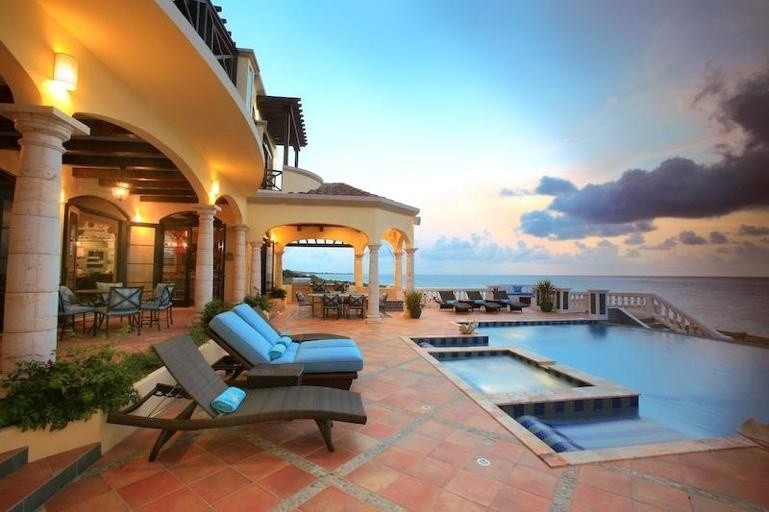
[53,52,79,91]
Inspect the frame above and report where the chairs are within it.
[439,290,529,314]
[296,280,389,320]
[203,302,366,390]
[107,333,365,461]
[58,281,177,340]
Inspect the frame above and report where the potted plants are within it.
[403,289,422,319]
[534,280,556,312]
